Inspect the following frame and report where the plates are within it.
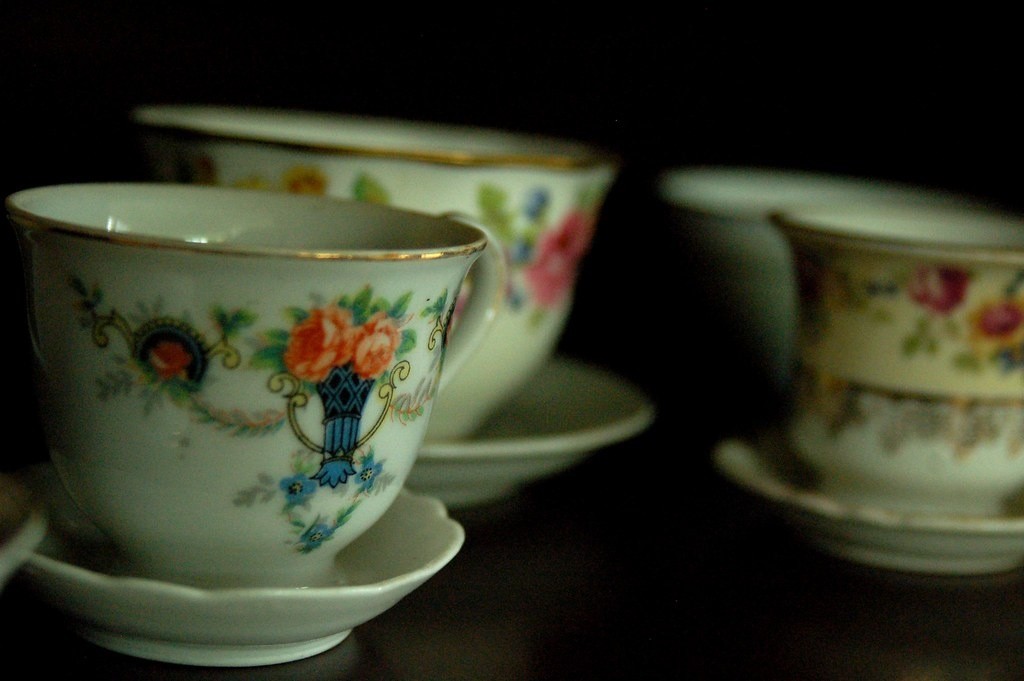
[406,355,655,506]
[16,484,465,666]
[713,441,1024,575]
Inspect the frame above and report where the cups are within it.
[769,205,1024,518]
[6,188,509,589]
[667,169,951,388]
[133,107,615,439]
